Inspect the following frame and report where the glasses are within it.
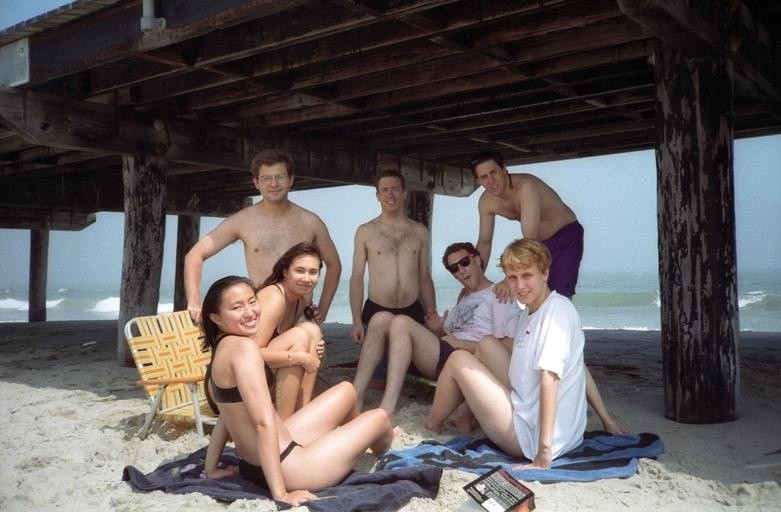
[448,255,471,272]
[256,172,289,185]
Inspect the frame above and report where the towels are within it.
[120,443,444,511]
[376,425,666,483]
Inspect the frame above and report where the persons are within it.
[349,170,436,380]
[183,150,342,326]
[198,276,399,506]
[204,241,326,420]
[423,237,627,470]
[457,149,584,298]
[353,242,523,415]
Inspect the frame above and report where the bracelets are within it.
[288,353,291,369]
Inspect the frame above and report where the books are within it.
[459,464,537,512]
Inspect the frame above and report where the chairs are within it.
[125,309,233,441]
